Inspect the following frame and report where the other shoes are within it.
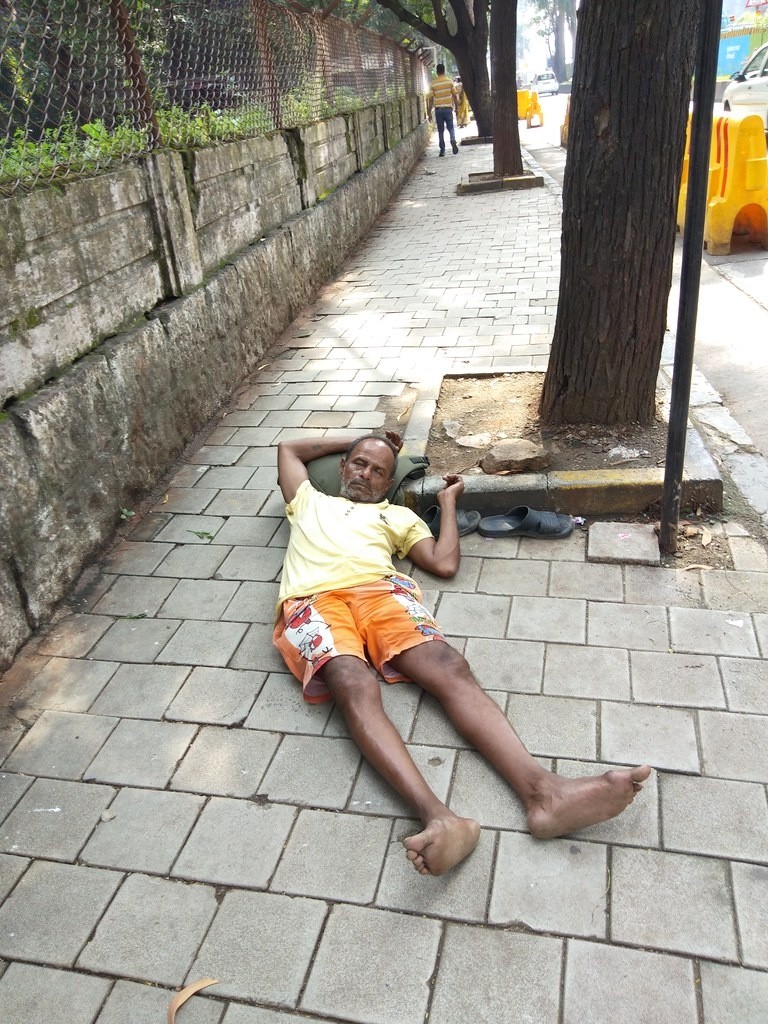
[452,144,458,154]
[439,151,445,156]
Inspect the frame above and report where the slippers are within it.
[478,505,575,540]
[419,506,480,542]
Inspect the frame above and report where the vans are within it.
[722,42,768,151]
[531,71,560,97]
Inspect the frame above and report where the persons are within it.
[453,77,469,128]
[427,64,458,157]
[270,431,650,878]
